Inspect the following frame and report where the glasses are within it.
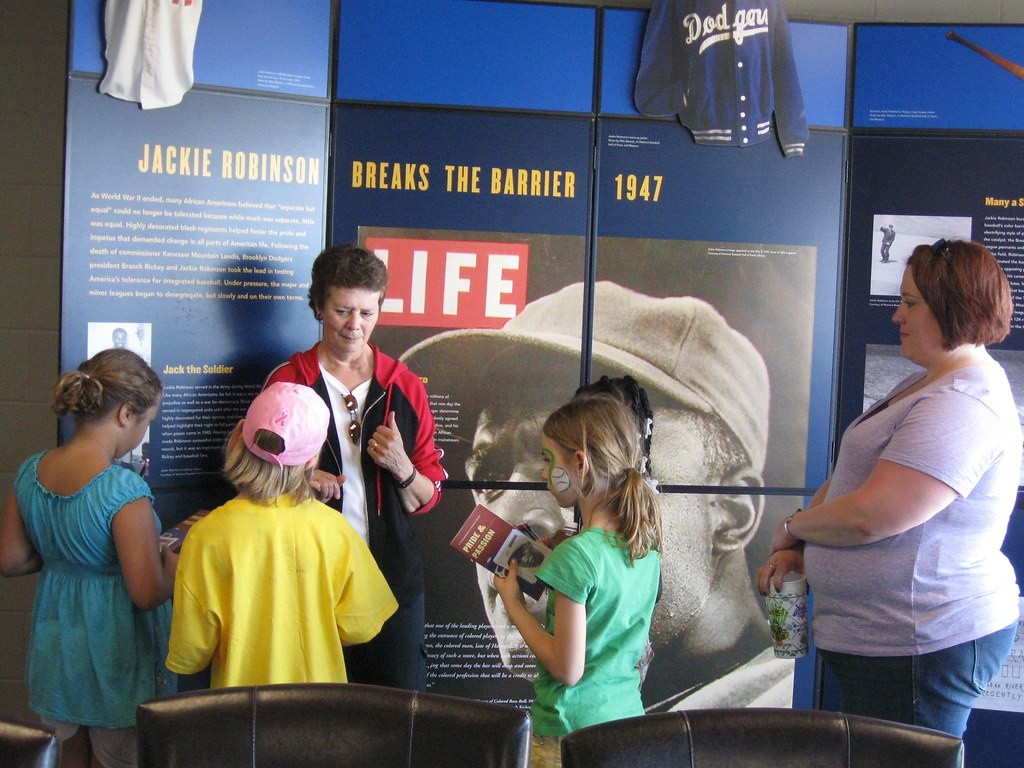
[341,392,361,444]
[932,238,952,266]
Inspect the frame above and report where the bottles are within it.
[553,522,578,548]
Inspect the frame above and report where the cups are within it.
[113,457,144,477]
[765,574,808,658]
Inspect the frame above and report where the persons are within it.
[1,348,180,768]
[756,240,1023,768]
[397,281,795,713]
[112,328,127,348]
[262,247,450,690]
[493,397,662,768]
[880,224,895,263]
[165,382,399,690]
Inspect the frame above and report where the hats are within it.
[397,280,771,476]
[242,382,330,471]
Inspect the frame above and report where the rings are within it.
[371,442,380,451]
[766,563,776,570]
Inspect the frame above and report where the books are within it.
[159,511,211,563]
[449,504,552,601]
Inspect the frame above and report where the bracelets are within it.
[396,464,416,488]
[784,508,803,539]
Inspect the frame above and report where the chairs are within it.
[560,706,961,768]
[131,681,534,768]
